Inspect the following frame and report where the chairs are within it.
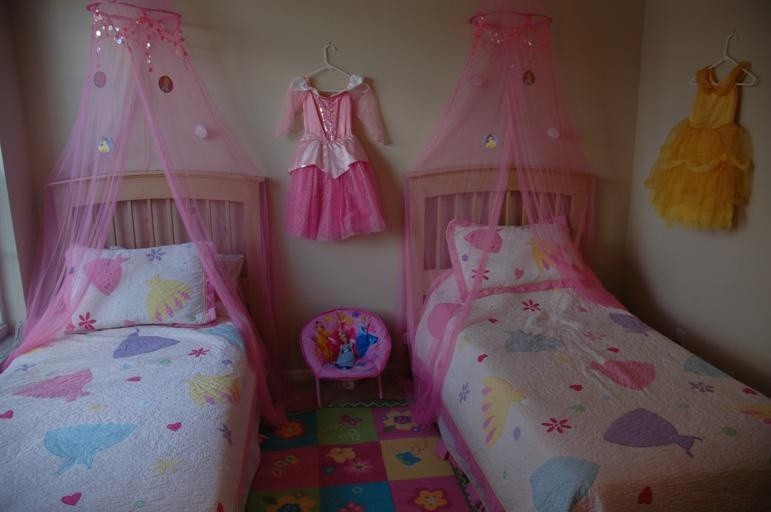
[299,309,390,407]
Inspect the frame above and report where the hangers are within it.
[687,30,761,90]
[290,43,372,94]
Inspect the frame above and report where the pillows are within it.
[62,236,245,337]
[446,218,591,297]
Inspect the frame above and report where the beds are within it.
[401,161,771,509]
[2,171,268,510]
